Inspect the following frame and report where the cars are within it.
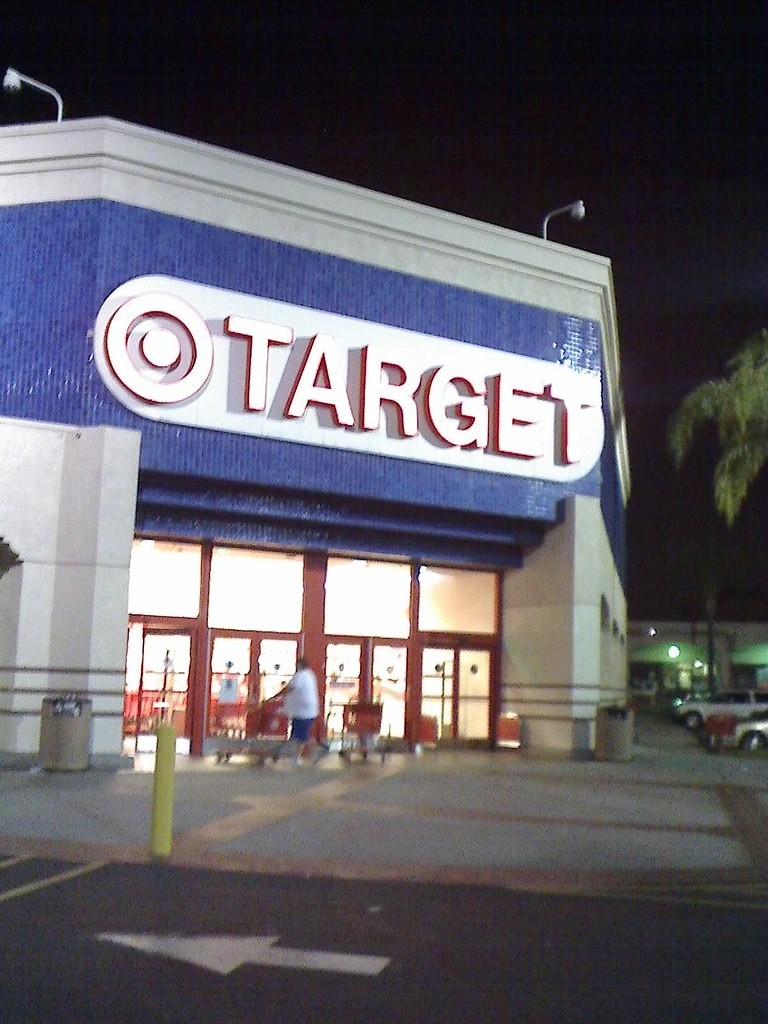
[674,689,768,731]
[712,717,768,753]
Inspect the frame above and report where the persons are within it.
[266,658,319,763]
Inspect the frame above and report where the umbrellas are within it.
[730,644,768,666]
[625,640,713,666]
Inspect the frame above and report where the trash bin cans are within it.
[38,695,93,770]
[594,704,636,761]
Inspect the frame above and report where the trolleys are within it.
[338,701,383,763]
[212,696,292,764]
